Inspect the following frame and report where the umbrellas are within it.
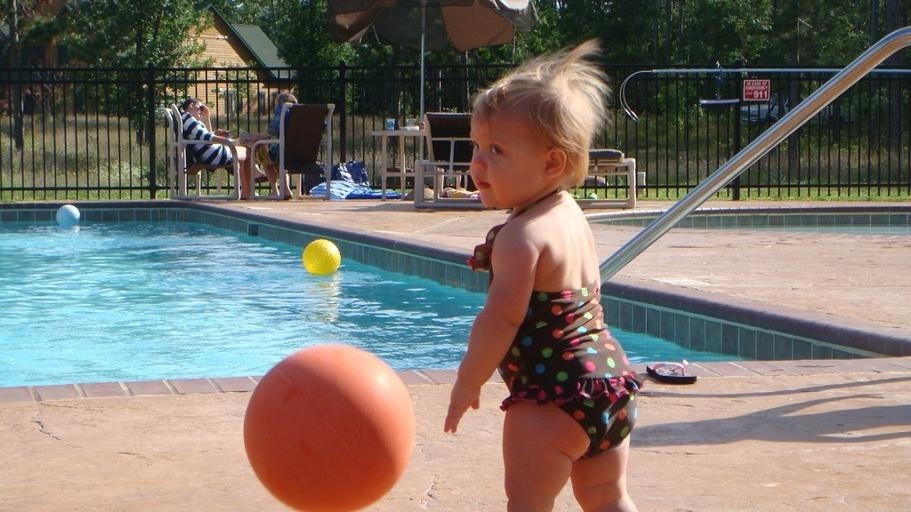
[325,0,541,160]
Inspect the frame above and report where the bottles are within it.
[588,193,597,199]
[573,191,579,199]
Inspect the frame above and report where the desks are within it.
[371,129,461,200]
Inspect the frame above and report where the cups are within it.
[384,118,395,130]
[405,118,417,126]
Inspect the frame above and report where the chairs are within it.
[413,111,483,207]
[250,103,336,200]
[574,148,636,209]
[163,103,240,201]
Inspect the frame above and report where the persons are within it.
[253,94,299,199]
[443,36,650,512]
[172,95,269,200]
